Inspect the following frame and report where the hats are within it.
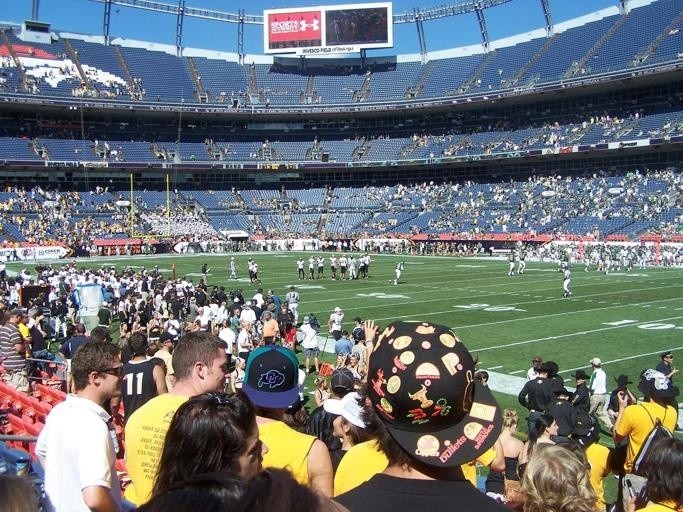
[242,345,301,410]
[335,307,341,311]
[572,414,592,436]
[222,298,253,307]
[367,320,505,470]
[352,317,361,321]
[332,368,356,392]
[534,350,672,396]
[161,332,179,344]
[324,391,372,430]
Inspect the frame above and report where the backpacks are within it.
[632,404,675,476]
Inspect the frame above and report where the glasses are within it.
[666,356,674,360]
[167,340,176,344]
[99,366,125,378]
[533,361,540,363]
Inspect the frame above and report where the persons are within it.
[332,319,517,510]
[520,437,604,511]
[123,331,229,511]
[611,369,678,512]
[332,438,478,498]
[0,45,683,475]
[35,341,127,512]
[149,387,270,502]
[0,473,39,512]
[484,407,524,502]
[237,343,333,499]
[626,437,683,512]
[133,463,351,511]
[572,411,613,511]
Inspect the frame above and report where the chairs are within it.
[1,368,129,497]
